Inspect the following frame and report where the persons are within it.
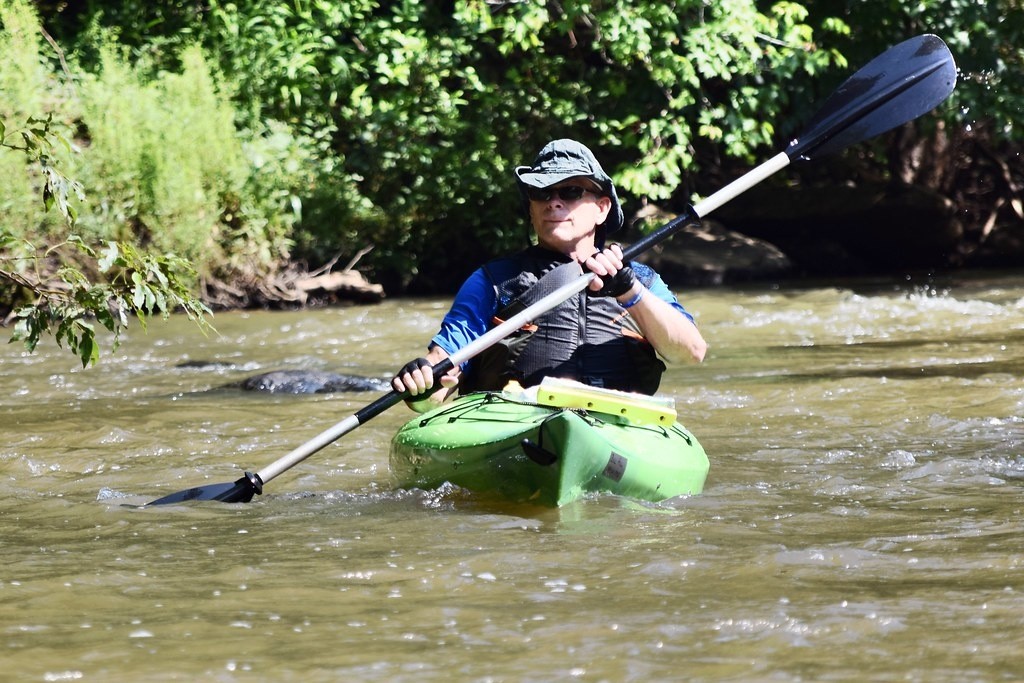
[391,139,706,416]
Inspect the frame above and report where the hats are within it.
[513,138,625,233]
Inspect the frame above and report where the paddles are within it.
[142,34,957,509]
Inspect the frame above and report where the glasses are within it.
[526,185,600,203]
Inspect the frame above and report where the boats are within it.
[386,371,711,519]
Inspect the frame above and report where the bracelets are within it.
[616,284,645,308]
